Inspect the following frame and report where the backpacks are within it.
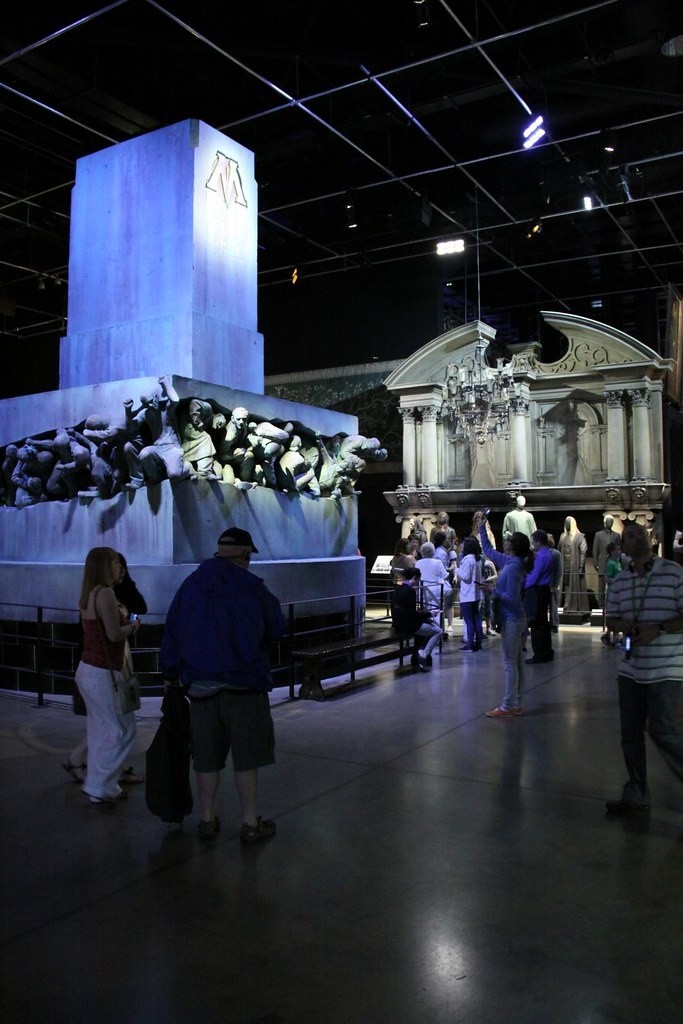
[146,685,194,829]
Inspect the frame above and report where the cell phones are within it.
[130,613,137,621]
[473,581,481,586]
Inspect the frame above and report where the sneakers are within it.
[457,645,482,653]
[241,817,276,845]
[197,814,219,847]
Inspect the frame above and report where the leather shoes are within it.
[526,657,545,665]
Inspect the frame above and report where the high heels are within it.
[79,790,128,813]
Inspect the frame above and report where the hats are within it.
[217,528,258,554]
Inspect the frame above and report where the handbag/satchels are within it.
[111,677,142,715]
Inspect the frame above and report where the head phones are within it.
[629,556,656,573]
[213,552,251,561]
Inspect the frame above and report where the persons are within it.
[406,496,620,612]
[478,509,534,719]
[672,528,683,554]
[0,376,388,509]
[61,545,148,806]
[525,530,564,666]
[605,522,683,825]
[390,568,444,673]
[396,532,498,654]
[601,542,624,647]
[158,526,286,846]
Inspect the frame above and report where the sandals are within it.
[117,767,144,785]
[62,757,88,783]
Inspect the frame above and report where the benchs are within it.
[293,628,419,701]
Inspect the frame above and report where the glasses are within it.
[507,537,512,544]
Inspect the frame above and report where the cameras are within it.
[482,509,490,520]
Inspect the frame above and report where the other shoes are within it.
[485,706,525,718]
[605,796,653,812]
[550,624,558,632]
[485,629,495,636]
